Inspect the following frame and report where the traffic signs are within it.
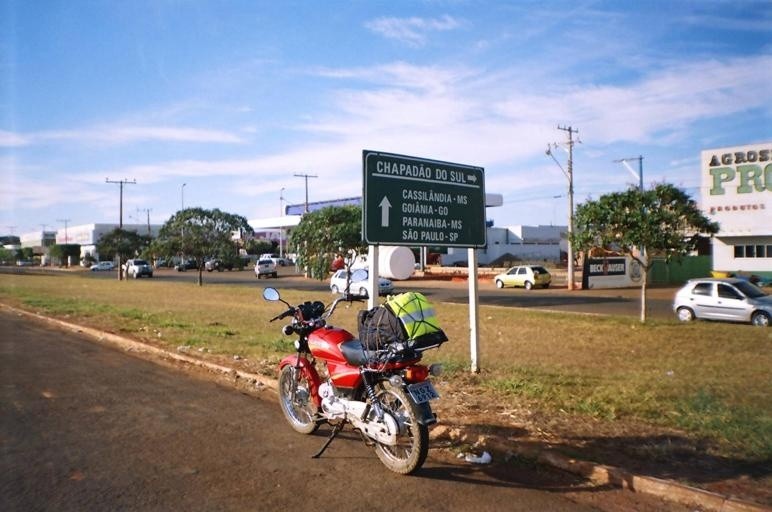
[359,148,488,251]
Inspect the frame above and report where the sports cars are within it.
[710,270,772,294]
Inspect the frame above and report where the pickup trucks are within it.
[205,255,251,272]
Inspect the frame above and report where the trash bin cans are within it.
[734,271,751,281]
[711,271,729,278]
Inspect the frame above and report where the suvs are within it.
[257,253,291,266]
[255,260,277,279]
[493,265,551,291]
[672,278,772,326]
[329,270,394,300]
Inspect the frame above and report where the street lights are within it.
[280,188,288,257]
[180,182,188,254]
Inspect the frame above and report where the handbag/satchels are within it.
[384,292,448,348]
[358,302,403,350]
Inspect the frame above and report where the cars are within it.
[16,258,33,267]
[89,255,197,279]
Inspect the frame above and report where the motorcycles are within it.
[261,268,450,476]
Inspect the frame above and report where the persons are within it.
[334,254,345,269]
